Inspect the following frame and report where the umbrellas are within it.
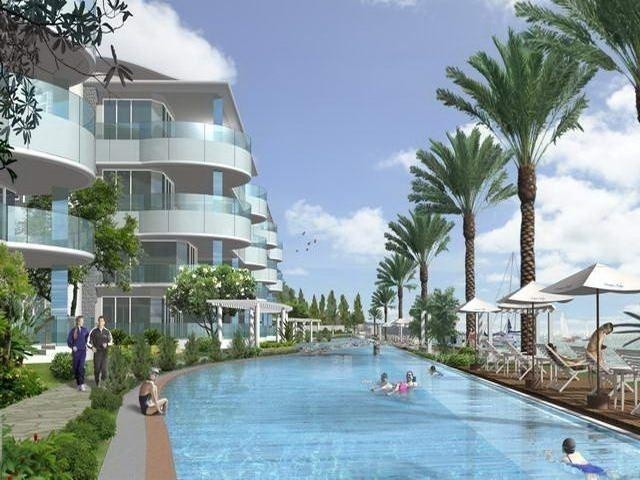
[383,319,395,328]
[457,280,575,375]
[539,262,640,393]
[392,318,411,342]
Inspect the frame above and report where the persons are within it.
[545,437,621,480]
[586,323,613,389]
[86,316,113,387]
[67,316,91,392]
[139,367,168,416]
[370,365,445,398]
[548,342,588,367]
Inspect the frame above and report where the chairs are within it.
[480,340,640,415]
